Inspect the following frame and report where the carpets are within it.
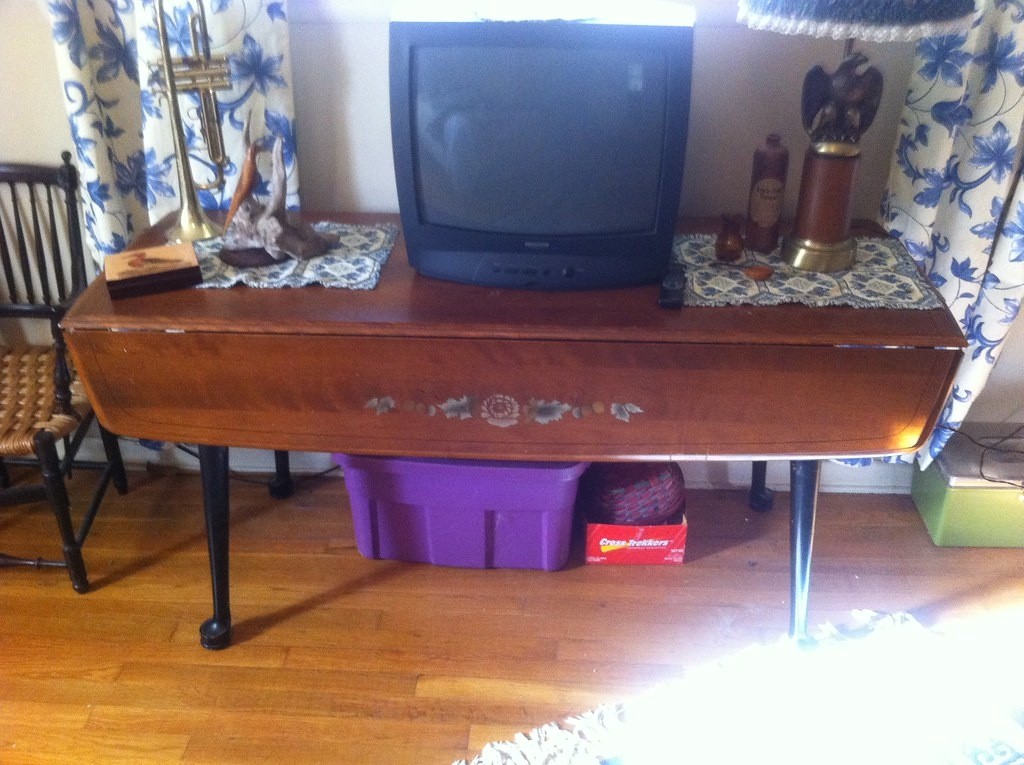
[443,608,1024,765]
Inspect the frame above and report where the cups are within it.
[718,213,752,261]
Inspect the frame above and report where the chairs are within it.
[0,161,130,593]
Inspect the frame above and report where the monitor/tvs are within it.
[389,0,696,294]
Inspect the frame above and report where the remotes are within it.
[659,262,689,308]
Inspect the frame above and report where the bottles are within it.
[745,133,788,253]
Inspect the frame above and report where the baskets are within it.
[592,457,686,526]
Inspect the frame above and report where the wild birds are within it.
[220,144,263,234]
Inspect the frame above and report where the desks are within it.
[62,207,969,643]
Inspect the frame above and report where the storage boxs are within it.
[579,493,687,564]
[910,422,1024,547]
[335,452,590,571]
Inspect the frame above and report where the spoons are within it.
[712,262,774,279]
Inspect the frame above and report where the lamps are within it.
[738,1,981,273]
